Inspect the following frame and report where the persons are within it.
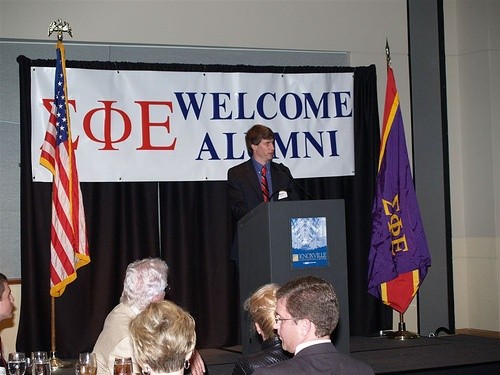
[84,256,206,375]
[251,275,374,375]
[231,283,293,375]
[227,125,299,275]
[131,301,196,375]
[0,273,15,375]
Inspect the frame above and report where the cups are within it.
[74,353,97,375]
[32,352,47,360]
[114,358,133,375]
[8,353,26,375]
[32,360,51,375]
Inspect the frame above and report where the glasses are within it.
[275,315,294,324]
[164,285,171,293]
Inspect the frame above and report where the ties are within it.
[261,166,269,201]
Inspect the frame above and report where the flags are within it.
[39,41,91,297]
[365,61,432,313]
